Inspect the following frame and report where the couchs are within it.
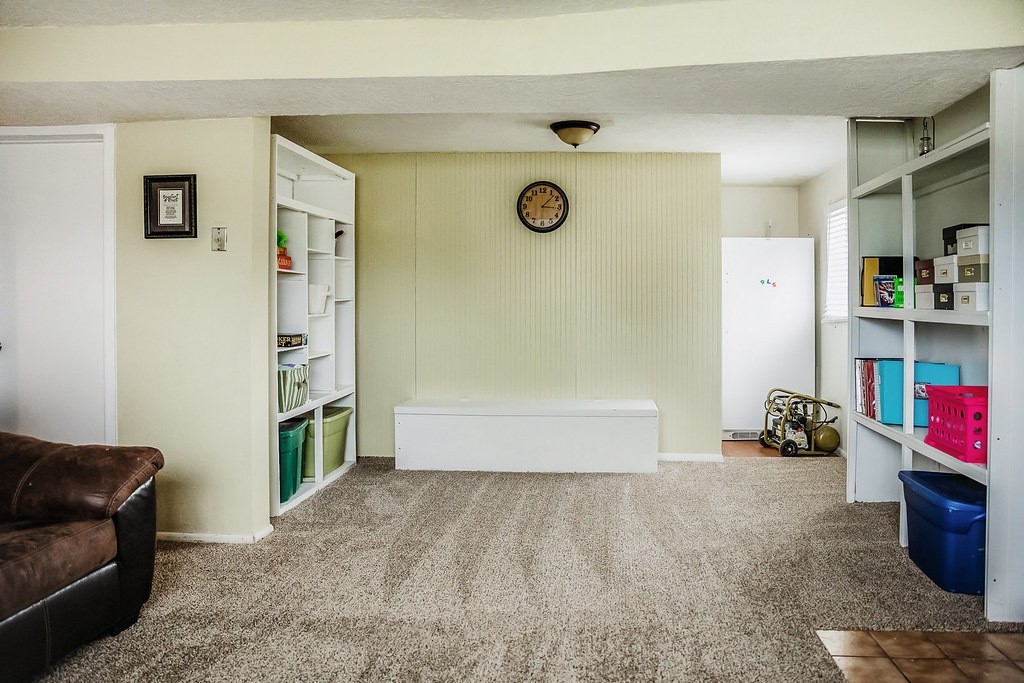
[0,429,164,683]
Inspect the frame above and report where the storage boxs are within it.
[912,224,989,314]
[295,406,353,477]
[280,417,308,503]
[898,471,985,596]
[924,384,988,463]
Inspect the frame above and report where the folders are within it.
[878,361,960,427]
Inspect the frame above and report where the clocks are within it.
[516,180,569,233]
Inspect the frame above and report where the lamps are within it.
[918,113,935,157]
[550,120,599,150]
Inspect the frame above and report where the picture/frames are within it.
[144,174,196,240]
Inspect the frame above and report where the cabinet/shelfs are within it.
[843,124,992,554]
[271,136,356,515]
